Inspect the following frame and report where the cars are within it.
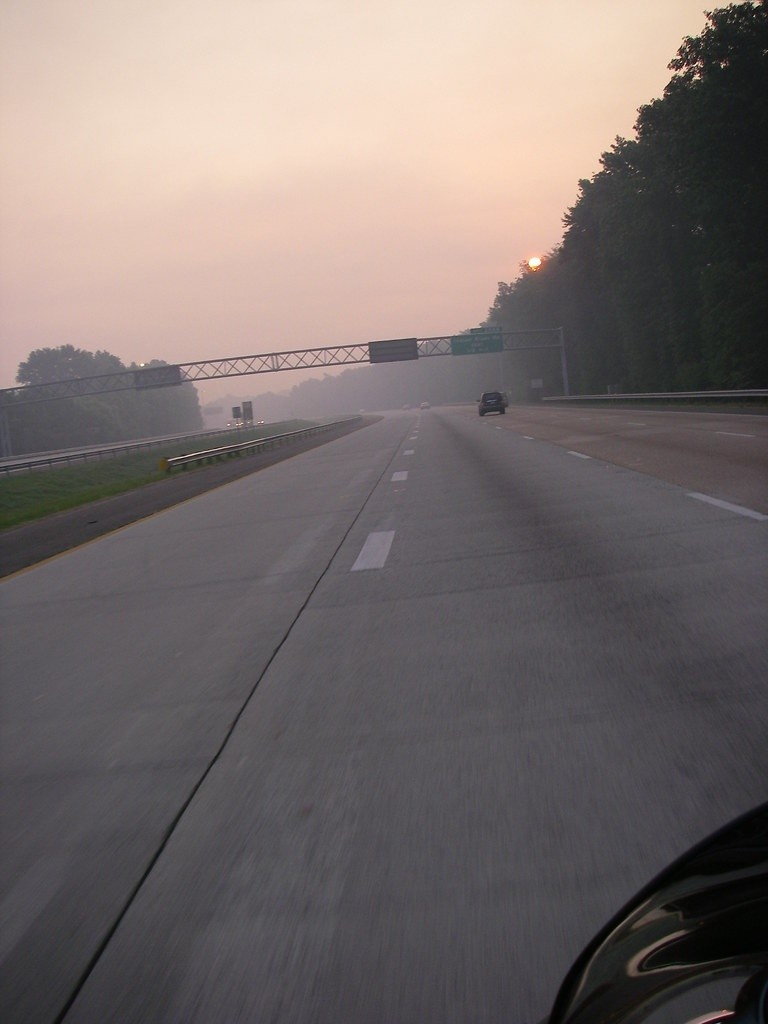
[477,392,510,416]
[419,402,431,410]
